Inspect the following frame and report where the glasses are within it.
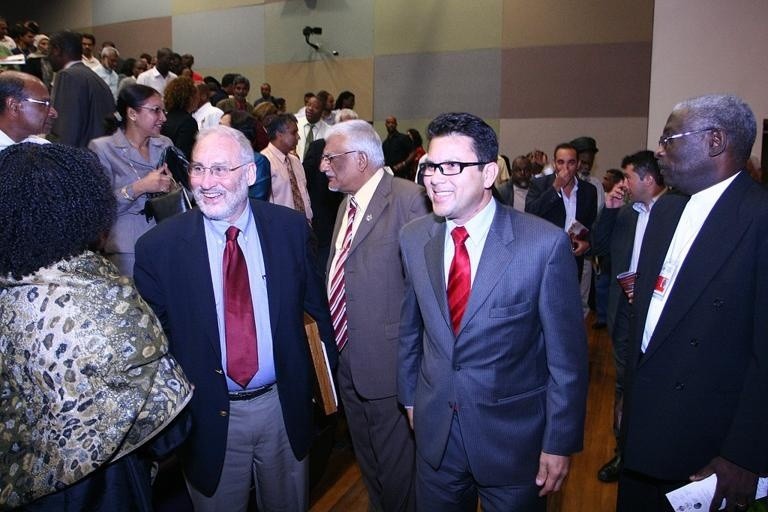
[418,158,492,176]
[18,95,50,109]
[185,162,248,179]
[321,150,362,165]
[659,125,718,150]
[135,105,168,115]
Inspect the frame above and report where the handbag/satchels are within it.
[149,174,193,225]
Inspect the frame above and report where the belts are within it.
[228,385,272,400]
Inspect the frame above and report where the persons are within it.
[395,112,588,512]
[494,137,627,330]
[1,18,430,512]
[615,94,767,512]
[596,151,672,483]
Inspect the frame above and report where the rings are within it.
[737,503,746,507]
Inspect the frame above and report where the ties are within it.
[329,195,358,352]
[303,123,315,162]
[222,228,259,390]
[447,227,474,336]
[284,156,306,217]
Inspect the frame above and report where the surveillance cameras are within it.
[303,26,322,36]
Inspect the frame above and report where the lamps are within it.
[302,25,322,50]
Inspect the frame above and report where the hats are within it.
[570,136,598,156]
[32,34,48,49]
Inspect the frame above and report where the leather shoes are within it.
[597,455,620,483]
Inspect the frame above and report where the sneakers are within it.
[591,319,607,330]
